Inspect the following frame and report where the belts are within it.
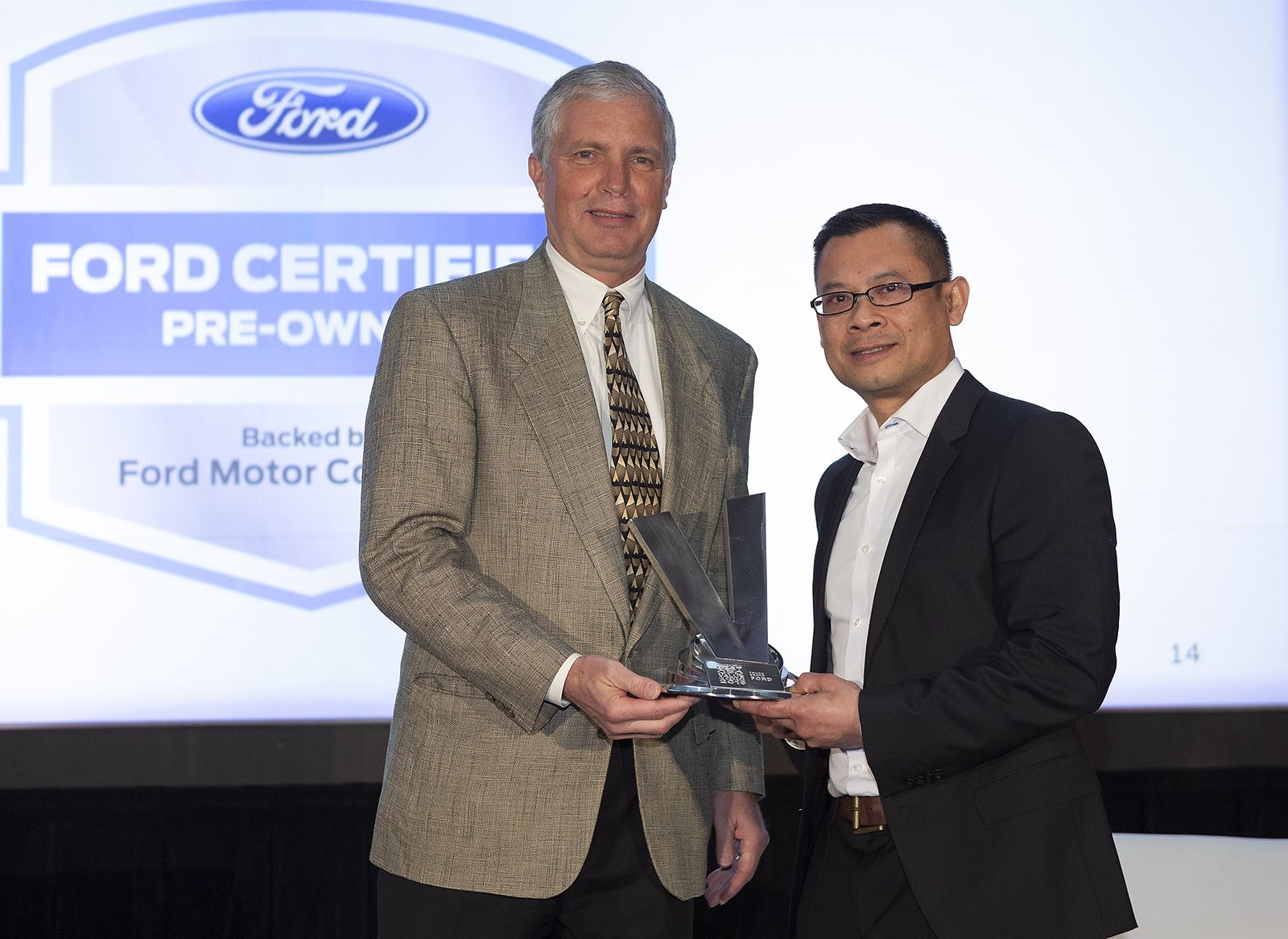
[826,793,888,835]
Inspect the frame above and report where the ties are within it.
[600,291,664,628]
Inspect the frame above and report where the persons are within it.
[734,204,1139,939]
[358,62,770,939]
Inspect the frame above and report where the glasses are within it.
[811,278,950,316]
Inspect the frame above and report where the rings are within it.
[736,856,740,860]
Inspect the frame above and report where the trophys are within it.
[626,492,815,697]
